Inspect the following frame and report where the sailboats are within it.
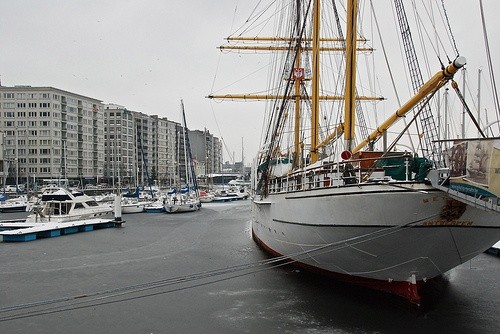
[0,98,251,242]
[204,0,500,307]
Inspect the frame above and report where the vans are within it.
[0,185,19,192]
